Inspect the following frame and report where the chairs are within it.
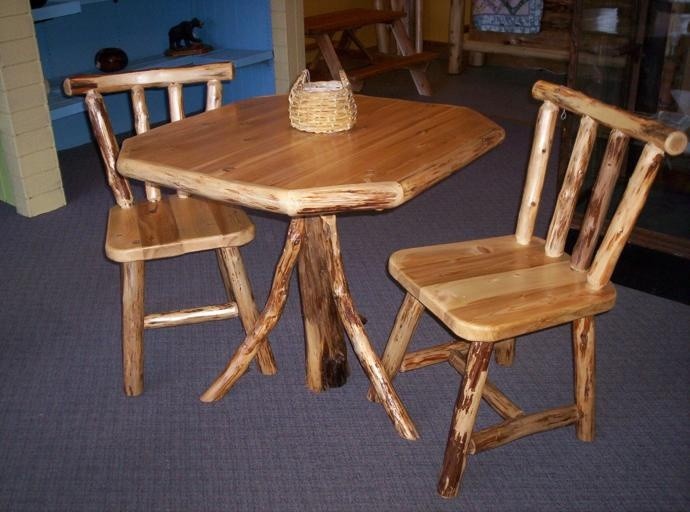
[63,63,279,397]
[366,79,688,500]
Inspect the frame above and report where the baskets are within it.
[288,70,357,135]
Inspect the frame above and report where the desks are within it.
[116,93,505,440]
[304,7,441,97]
